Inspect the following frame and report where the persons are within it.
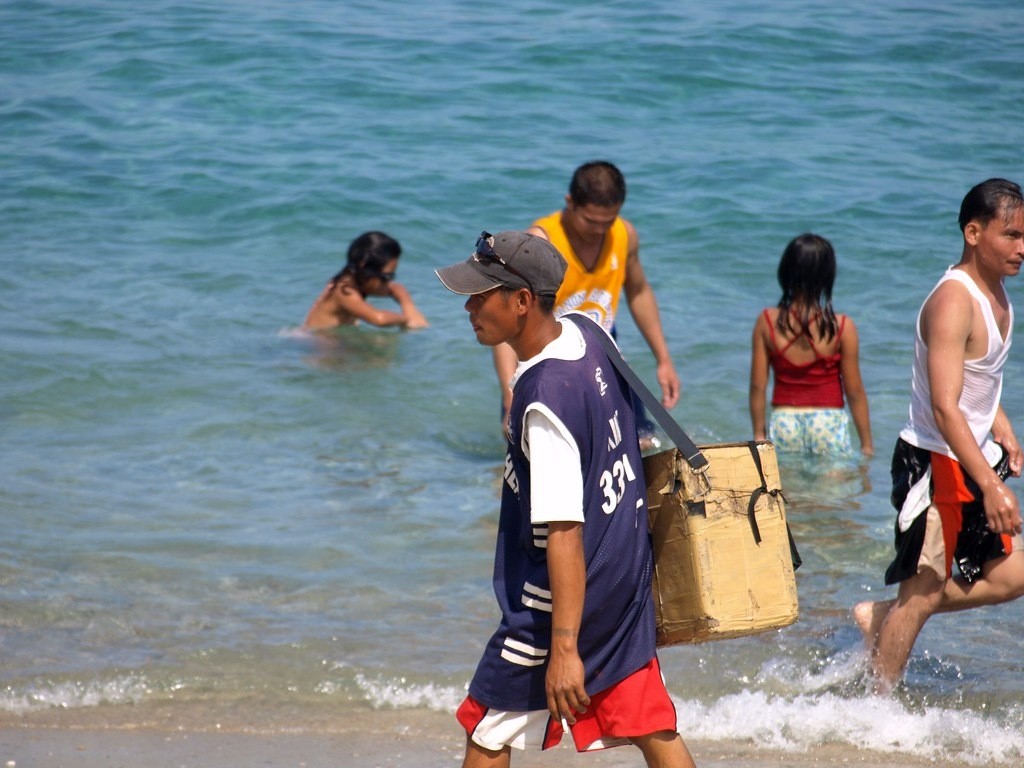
[491,160,681,455]
[749,233,875,455]
[855,177,1024,691]
[432,230,698,768]
[305,230,429,330]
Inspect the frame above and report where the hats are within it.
[434,231,568,296]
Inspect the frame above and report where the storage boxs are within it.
[642,439,799,648]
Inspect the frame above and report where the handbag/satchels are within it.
[571,314,799,648]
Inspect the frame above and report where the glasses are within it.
[376,272,394,282]
[475,230,536,302]
